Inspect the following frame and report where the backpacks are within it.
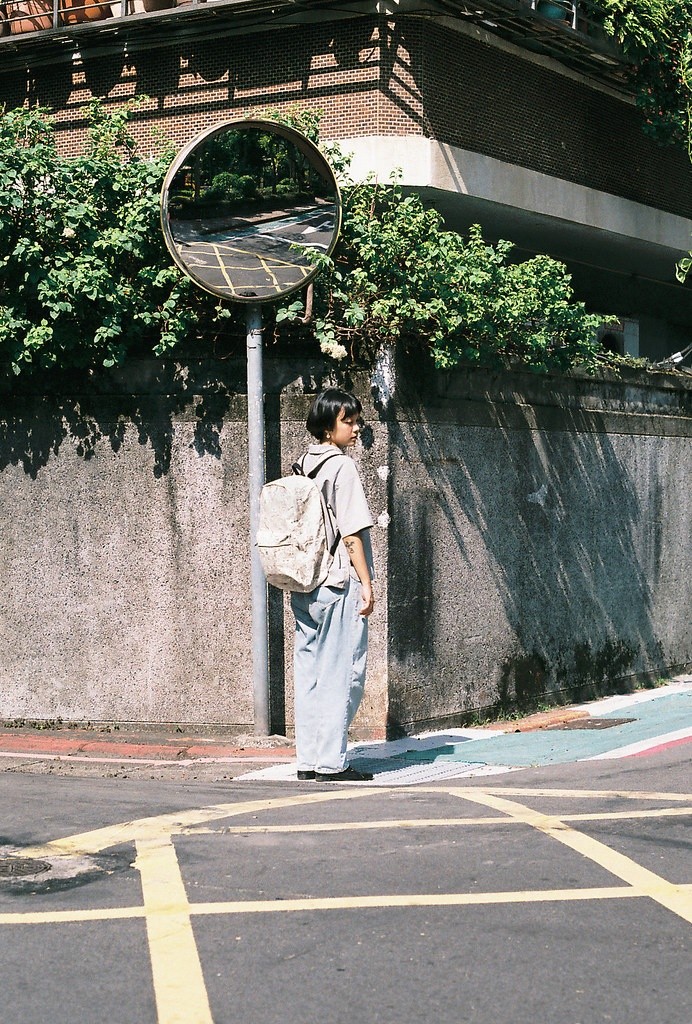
[259,452,351,595]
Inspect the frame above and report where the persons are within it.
[289,387,374,782]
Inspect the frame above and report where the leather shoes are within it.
[297,767,374,782]
